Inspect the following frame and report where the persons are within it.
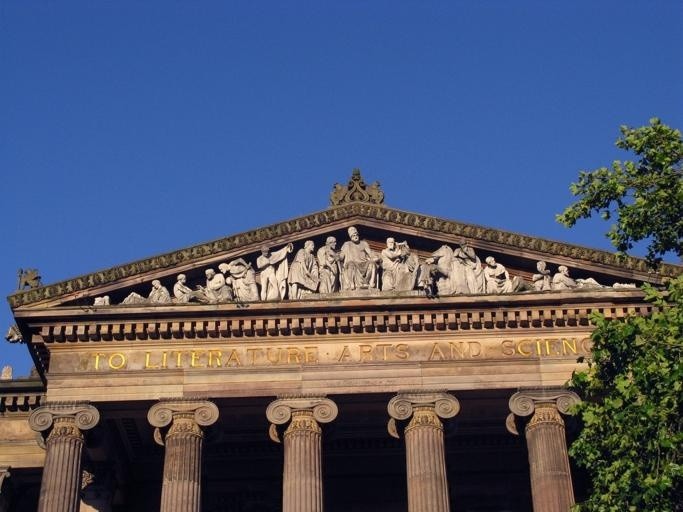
[149,227,449,306]
[16,268,44,293]
[454,239,580,295]
